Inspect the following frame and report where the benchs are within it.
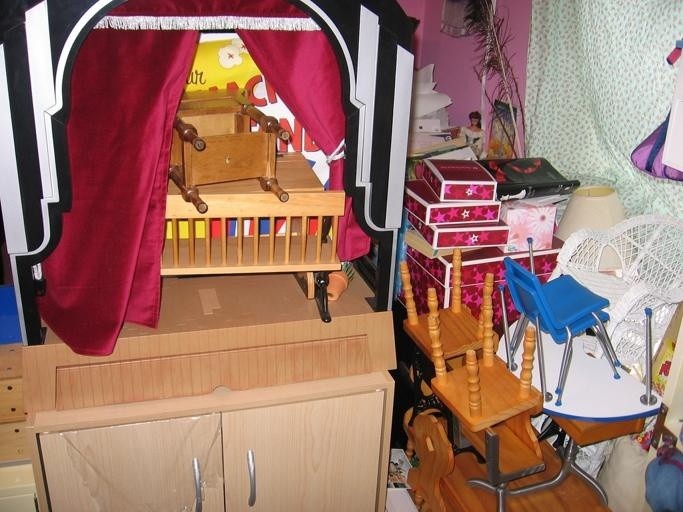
[156,190,347,324]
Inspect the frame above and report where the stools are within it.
[166,89,289,214]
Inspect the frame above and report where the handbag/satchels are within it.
[592,432,683,512]
[630,105,683,184]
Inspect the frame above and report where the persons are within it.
[458,111,485,161]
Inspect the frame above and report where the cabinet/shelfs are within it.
[26,372,395,512]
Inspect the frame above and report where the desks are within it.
[534,411,644,506]
[498,237,661,421]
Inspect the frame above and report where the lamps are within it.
[555,186,640,273]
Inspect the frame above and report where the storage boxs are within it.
[396,158,565,329]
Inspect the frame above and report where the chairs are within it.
[398,236,620,512]
